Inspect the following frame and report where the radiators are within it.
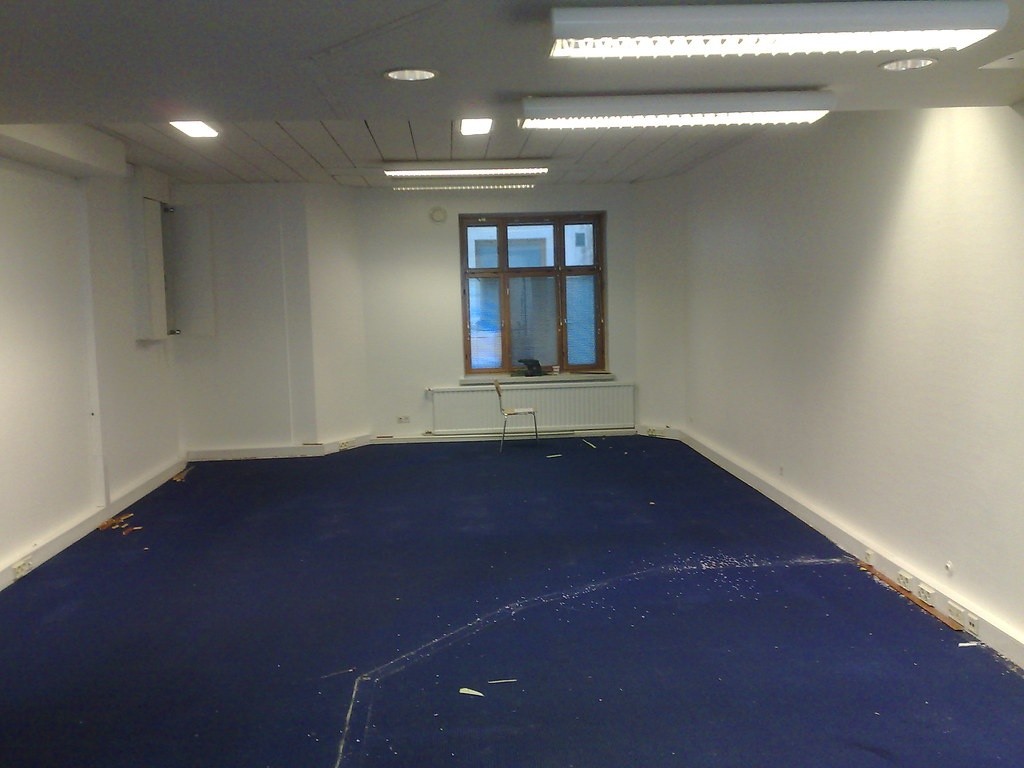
[425,380,636,435]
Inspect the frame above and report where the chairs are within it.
[493,378,539,453]
